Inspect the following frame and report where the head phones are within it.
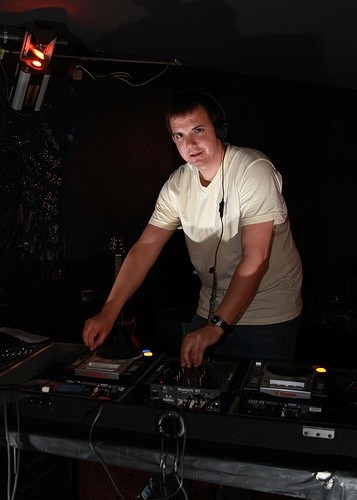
[174,90,229,141]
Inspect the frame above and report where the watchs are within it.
[208,313,229,333]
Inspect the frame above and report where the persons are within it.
[82,88,305,368]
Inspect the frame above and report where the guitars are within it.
[108,236,138,346]
